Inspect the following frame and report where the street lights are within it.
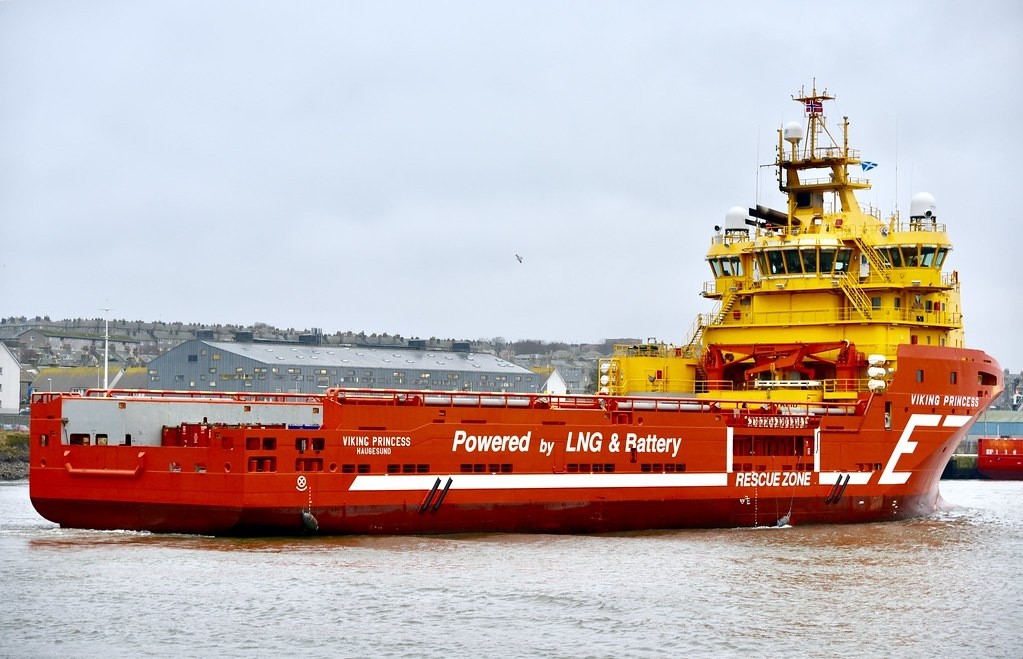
[98,308,116,398]
[48,379,51,392]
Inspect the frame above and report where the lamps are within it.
[912,281,921,285]
[730,287,738,292]
[830,280,841,286]
[776,283,786,289]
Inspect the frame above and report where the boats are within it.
[28,73,1005,541]
[977,434,1023,480]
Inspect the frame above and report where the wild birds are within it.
[515,254,522,263]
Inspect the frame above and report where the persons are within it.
[742,403,748,408]
[761,401,774,409]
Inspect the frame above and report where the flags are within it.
[806,100,823,112]
[862,162,878,172]
